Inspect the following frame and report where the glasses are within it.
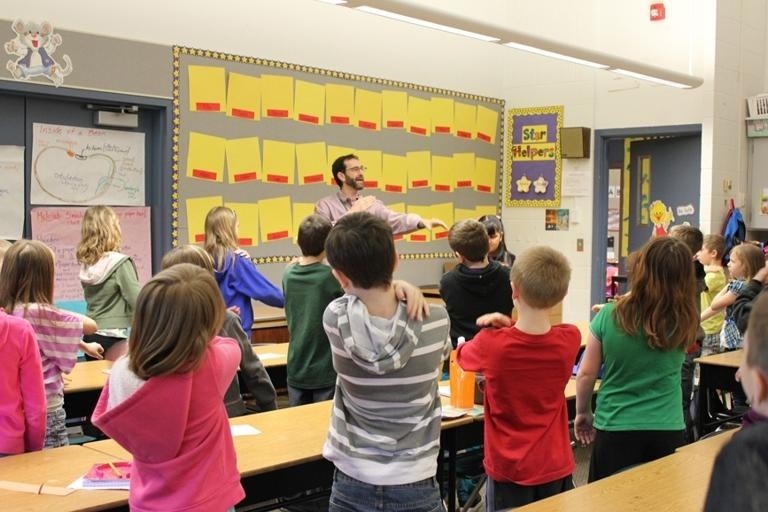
[345,166,367,172]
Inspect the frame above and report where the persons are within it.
[457,247,582,511]
[440,217,515,351]
[77,208,143,437]
[162,207,287,417]
[1,239,104,457]
[282,212,452,509]
[669,224,768,512]
[91,262,246,512]
[312,153,449,236]
[573,235,700,483]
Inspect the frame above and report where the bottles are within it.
[448,337,475,408]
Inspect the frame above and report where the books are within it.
[84,461,132,486]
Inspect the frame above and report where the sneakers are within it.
[458,494,482,512]
